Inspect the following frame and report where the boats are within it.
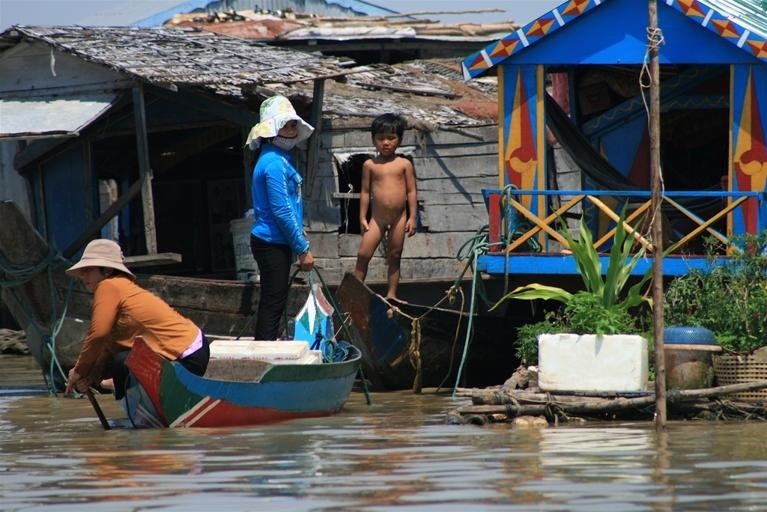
[117,325,365,432]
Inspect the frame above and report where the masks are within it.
[271,133,299,152]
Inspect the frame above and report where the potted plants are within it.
[487,190,766,405]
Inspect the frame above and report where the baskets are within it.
[661,324,717,346]
[712,345,767,403]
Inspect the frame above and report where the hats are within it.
[243,95,315,151]
[64,239,136,282]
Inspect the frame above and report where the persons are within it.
[241,94,315,342]
[60,236,210,394]
[354,110,420,298]
